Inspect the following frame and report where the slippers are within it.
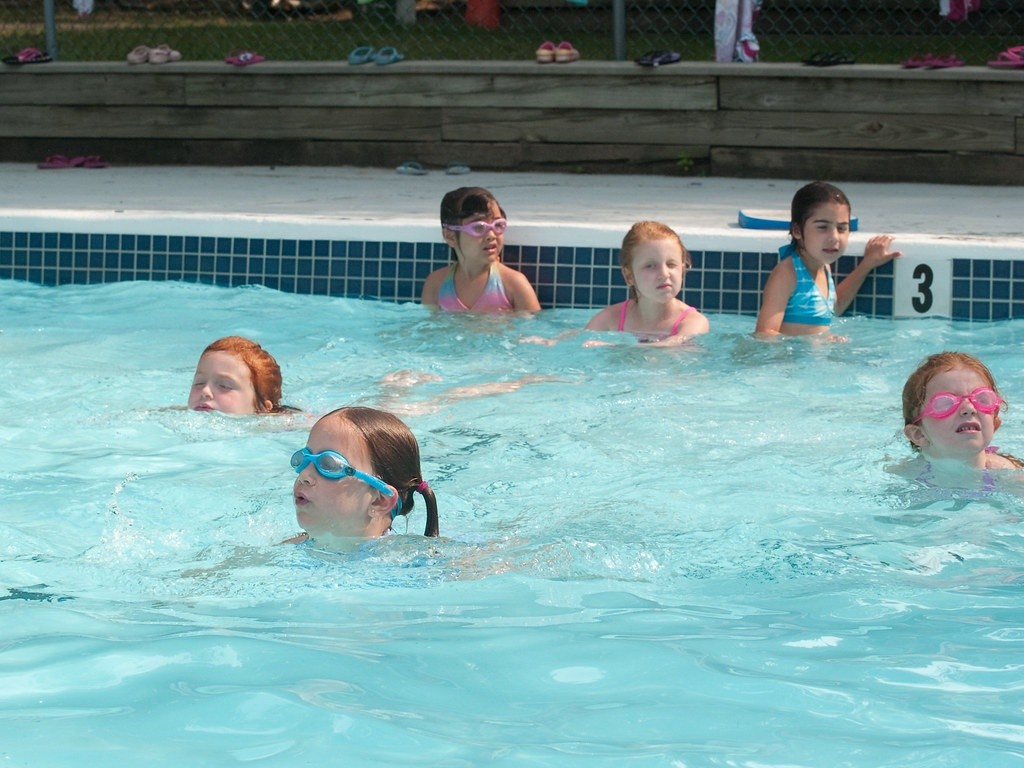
[227,50,264,65]
[38,154,108,168]
[801,51,856,67]
[397,161,428,175]
[349,46,403,65]
[635,49,682,67]
[900,53,963,67]
[536,41,580,62]
[2,47,52,64]
[128,44,181,64]
[987,46,1024,69]
[445,162,470,174]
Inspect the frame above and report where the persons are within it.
[753,179,900,349]
[138,333,565,416]
[422,185,541,333]
[886,351,1024,524]
[519,220,709,362]
[180,403,556,583]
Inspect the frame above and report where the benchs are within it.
[0,59,1024,188]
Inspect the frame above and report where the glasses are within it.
[911,387,1002,424]
[291,447,402,521]
[443,219,507,237]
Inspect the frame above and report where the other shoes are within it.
[737,33,760,63]
